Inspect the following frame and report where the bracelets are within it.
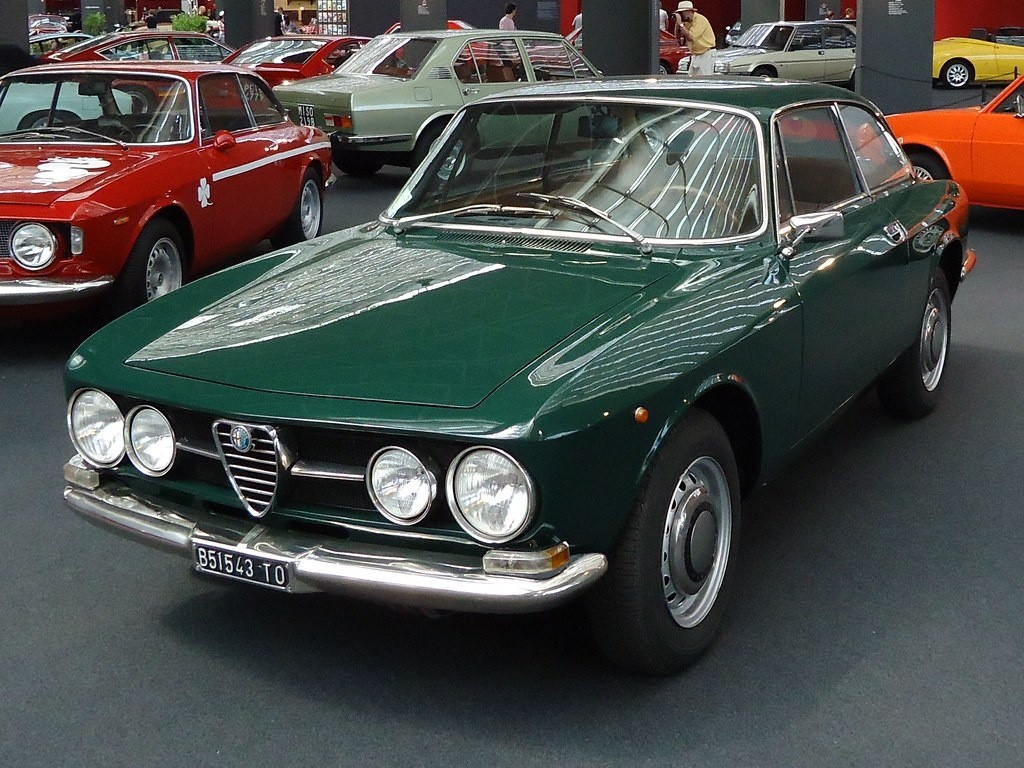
[679,24,684,28]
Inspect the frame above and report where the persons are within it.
[842,8,857,20]
[218,10,225,44]
[274,6,300,36]
[572,4,582,30]
[659,1,668,32]
[499,2,517,30]
[671,1,716,76]
[824,10,835,20]
[198,6,206,19]
[145,10,157,29]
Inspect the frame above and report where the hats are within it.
[674,1,697,12]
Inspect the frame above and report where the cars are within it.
[513,21,690,76]
[677,19,859,93]
[60,69,980,676]
[267,26,610,192]
[856,68,1024,220]
[40,29,239,68]
[0,64,339,314]
[27,13,95,57]
[187,33,415,124]
[931,25,1024,88]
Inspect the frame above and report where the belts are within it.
[710,47,716,49]
